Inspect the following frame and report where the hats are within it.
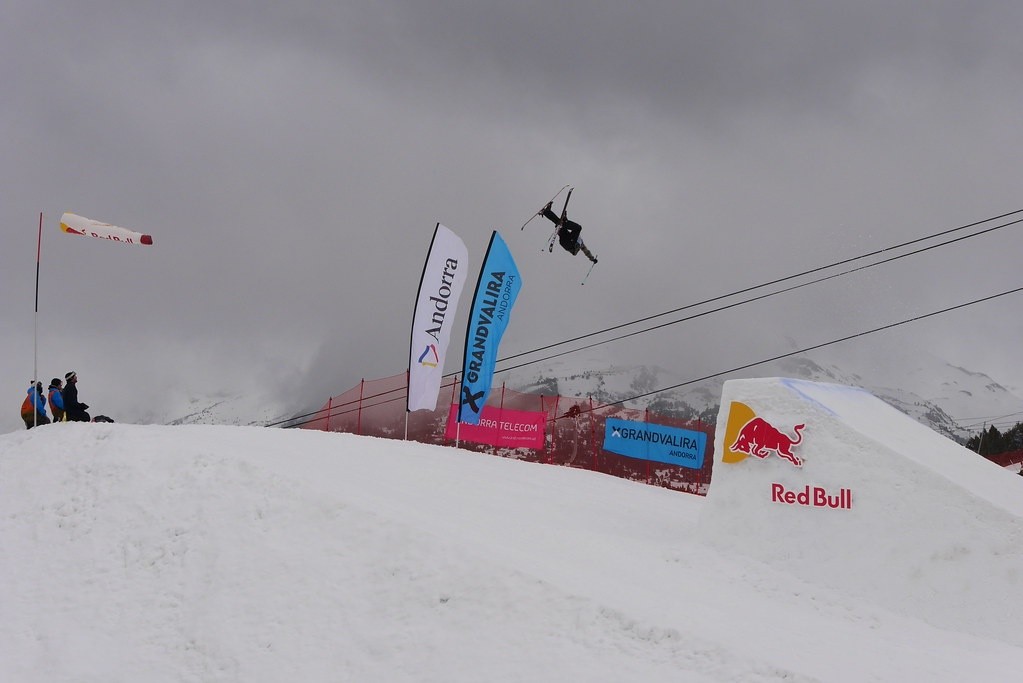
[65,372,76,380]
[51,378,61,386]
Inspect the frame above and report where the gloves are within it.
[592,259,598,264]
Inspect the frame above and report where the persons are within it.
[21,380,51,430]
[63,371,90,422]
[542,202,598,264]
[48,378,66,423]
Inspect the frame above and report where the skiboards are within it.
[521,184,573,253]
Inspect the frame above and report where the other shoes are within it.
[543,205,552,215]
[563,211,567,222]
